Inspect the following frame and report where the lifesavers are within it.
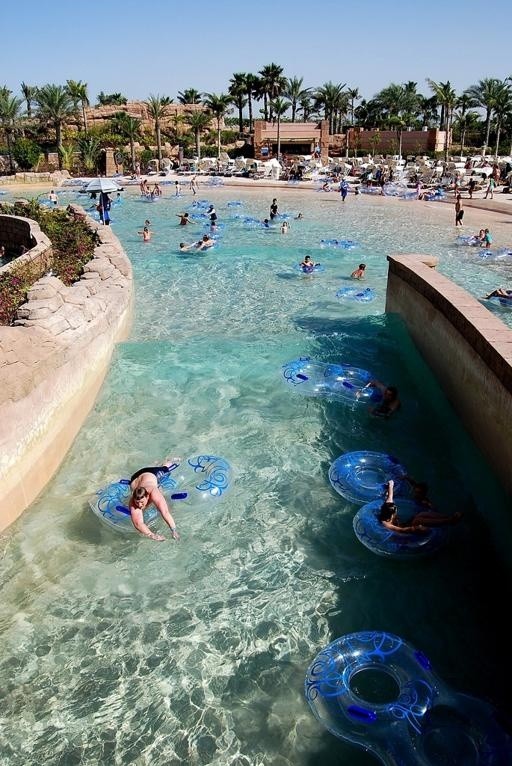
[40,176,223,221]
[184,199,299,251]
[158,456,234,507]
[305,632,512,765]
[88,479,166,534]
[488,295,512,307]
[292,264,324,272]
[456,236,481,247]
[353,498,451,559]
[319,239,361,250]
[479,247,511,259]
[357,182,446,201]
[280,356,384,408]
[335,287,377,305]
[329,451,414,505]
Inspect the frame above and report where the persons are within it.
[48,159,223,252]
[356,380,401,417]
[381,476,464,534]
[264,199,304,234]
[455,195,464,226]
[300,255,321,269]
[485,288,512,299]
[279,151,512,201]
[466,229,492,248]
[351,263,367,278]
[129,462,179,541]
